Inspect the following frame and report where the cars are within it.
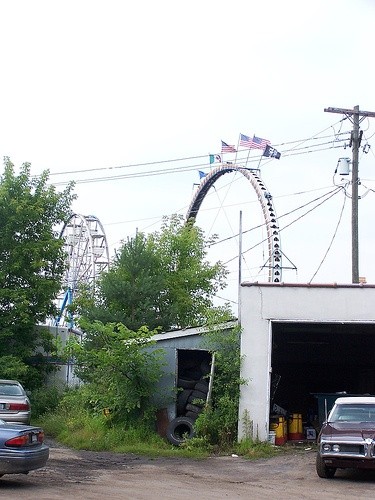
[0,418,49,479]
[0,380,31,425]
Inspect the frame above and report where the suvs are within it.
[316,396,375,479]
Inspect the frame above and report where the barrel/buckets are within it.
[270,414,302,445]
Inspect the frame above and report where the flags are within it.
[263,145,281,159]
[239,134,271,151]
[200,171,207,179]
[221,141,237,153]
[210,155,221,163]
